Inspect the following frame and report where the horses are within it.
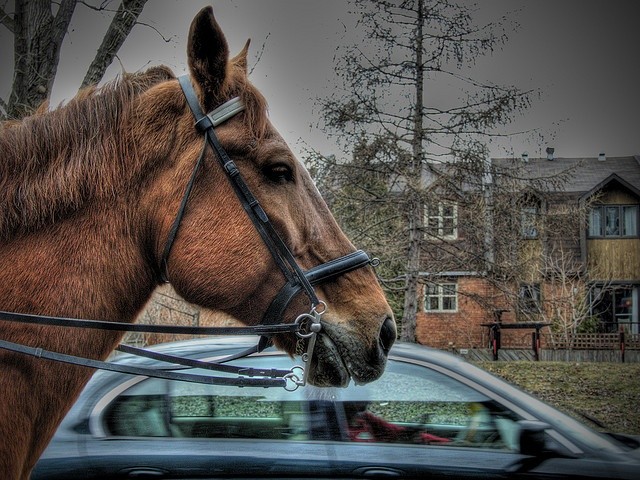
[0,5,398,480]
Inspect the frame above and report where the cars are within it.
[32,335,640,479]
